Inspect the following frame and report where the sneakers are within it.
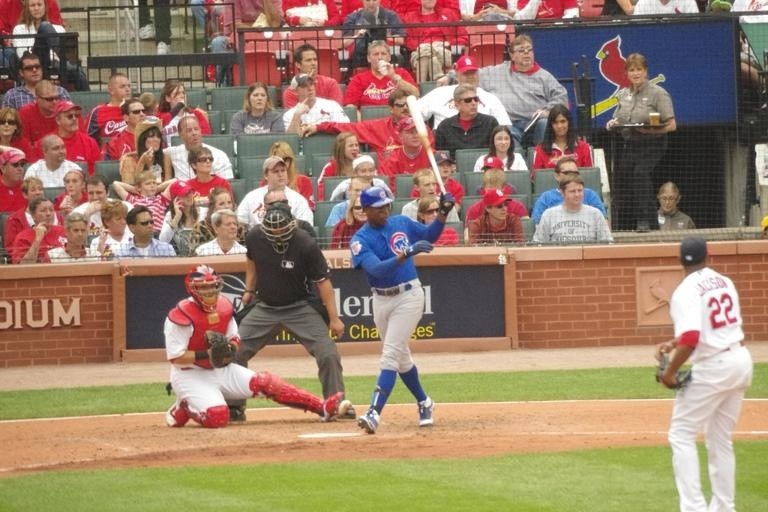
[320,392,343,423]
[120,24,154,40]
[417,396,434,426]
[230,407,246,421]
[166,400,187,427]
[158,42,170,55]
[358,409,380,434]
[340,399,356,419]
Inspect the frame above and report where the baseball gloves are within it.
[655,360,690,389]
[207,331,235,368]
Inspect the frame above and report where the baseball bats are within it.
[407,94,454,209]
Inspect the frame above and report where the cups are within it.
[377,60,387,75]
[64,199,74,213]
[649,112,661,125]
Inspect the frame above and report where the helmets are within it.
[260,203,298,254]
[360,186,394,209]
[185,263,224,313]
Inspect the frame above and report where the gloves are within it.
[403,239,434,257]
[439,192,455,216]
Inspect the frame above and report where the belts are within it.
[375,283,411,297]
[723,339,747,352]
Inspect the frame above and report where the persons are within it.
[221,200,358,424]
[604,0,768,230]
[161,264,344,430]
[190,0,615,247]
[655,235,756,511]
[349,187,457,434]
[0,0,248,262]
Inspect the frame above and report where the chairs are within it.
[1,0,614,262]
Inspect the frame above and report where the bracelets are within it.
[244,290,257,294]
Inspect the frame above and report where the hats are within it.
[291,73,314,91]
[456,55,479,73]
[136,121,162,138]
[681,236,707,266]
[484,189,512,207]
[430,150,456,165]
[481,157,504,171]
[263,156,287,176]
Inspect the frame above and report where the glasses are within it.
[422,207,440,215]
[394,101,408,108]
[136,219,155,226]
[144,130,163,138]
[461,96,480,104]
[492,202,509,209]
[561,170,580,176]
[512,45,534,53]
[190,156,214,164]
[129,109,148,115]
[0,63,81,167]
